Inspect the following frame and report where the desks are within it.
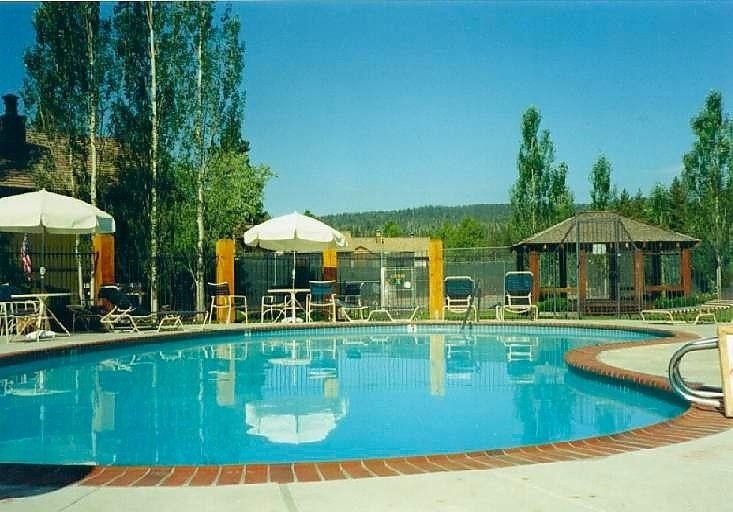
[267,288,311,324]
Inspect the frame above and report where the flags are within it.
[20,232,32,282]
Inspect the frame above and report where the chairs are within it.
[260,283,295,324]
[640,297,733,326]
[97,286,153,334]
[155,303,207,333]
[496,270,539,322]
[208,282,249,324]
[305,281,338,324]
[1,284,106,338]
[440,274,479,324]
[331,284,366,323]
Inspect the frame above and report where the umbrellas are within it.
[243,211,349,316]
[0,406,92,464]
[245,335,349,446]
[0,188,117,330]
